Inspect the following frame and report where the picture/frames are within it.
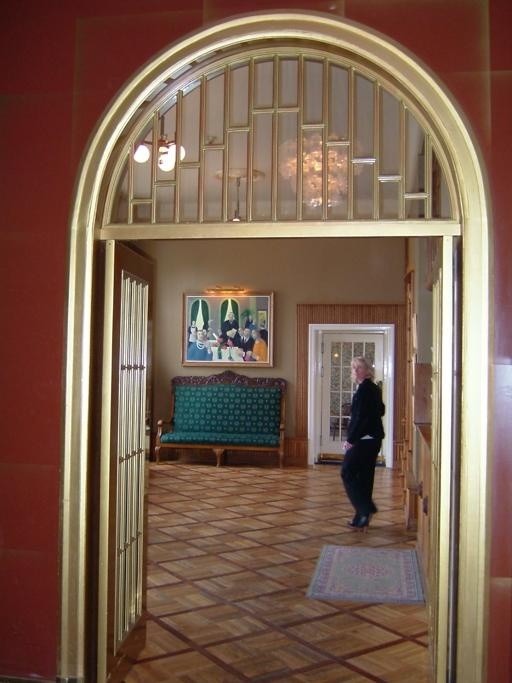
[180,290,275,369]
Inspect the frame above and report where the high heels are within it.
[348,502,377,528]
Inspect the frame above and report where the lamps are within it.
[214,167,265,222]
[134,116,186,172]
[278,132,364,210]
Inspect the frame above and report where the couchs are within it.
[155,370,288,469]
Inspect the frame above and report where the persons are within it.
[339,356,387,533]
[187,310,266,362]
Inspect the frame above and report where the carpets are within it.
[306,543,425,604]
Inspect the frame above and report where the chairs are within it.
[333,403,352,442]
